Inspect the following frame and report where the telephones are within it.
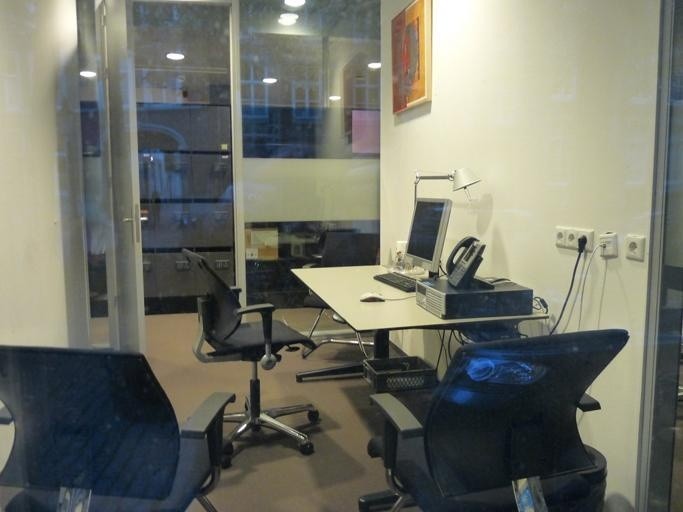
[446,235,486,290]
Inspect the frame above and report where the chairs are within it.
[355,328,630,512]
[0,342,237,512]
[181,247,321,469]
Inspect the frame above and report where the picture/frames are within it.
[392,0,433,115]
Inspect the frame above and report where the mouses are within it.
[359,292,384,302]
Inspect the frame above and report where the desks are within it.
[287,261,551,460]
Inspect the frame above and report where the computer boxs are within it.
[416,278,533,318]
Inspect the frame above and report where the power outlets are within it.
[576,228,593,251]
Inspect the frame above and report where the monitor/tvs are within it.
[403,198,452,273]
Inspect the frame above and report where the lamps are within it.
[413,162,483,213]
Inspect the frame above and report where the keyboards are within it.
[374,271,416,292]
